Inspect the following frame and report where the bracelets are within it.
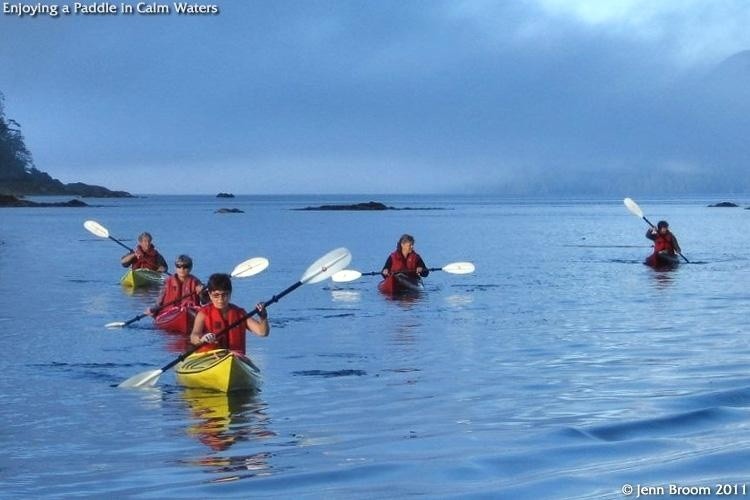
[259,310,268,318]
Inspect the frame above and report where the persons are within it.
[644,220,682,255]
[146,254,210,316]
[189,272,271,356]
[379,234,429,279]
[121,231,169,274]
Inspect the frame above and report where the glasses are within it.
[176,264,188,268]
[211,293,229,298]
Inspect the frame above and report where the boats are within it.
[645,252,679,267]
[120,268,263,393]
[377,274,421,295]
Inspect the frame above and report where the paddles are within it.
[117,248,351,388]
[105,258,269,328]
[332,262,474,282]
[624,197,689,263]
[84,220,172,276]
